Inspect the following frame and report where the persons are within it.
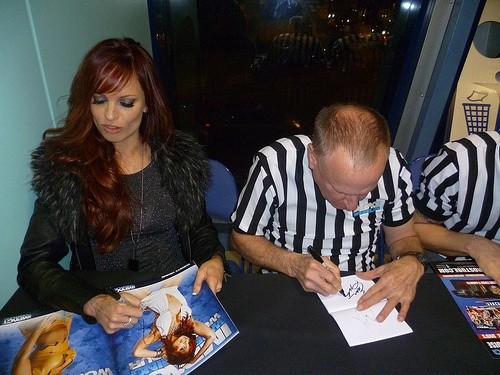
[16,38,228,333]
[230,101,426,323]
[413,130,500,287]
[132,272,216,367]
[12,316,77,375]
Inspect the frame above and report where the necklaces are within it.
[129,139,143,270]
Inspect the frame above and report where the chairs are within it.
[203,159,254,276]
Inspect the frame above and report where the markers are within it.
[102,285,132,307]
[307,245,347,298]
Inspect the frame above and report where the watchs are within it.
[395,251,429,272]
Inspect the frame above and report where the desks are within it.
[0,271,500,375]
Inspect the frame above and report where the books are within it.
[1,261,240,375]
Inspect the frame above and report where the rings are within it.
[124,316,131,326]
